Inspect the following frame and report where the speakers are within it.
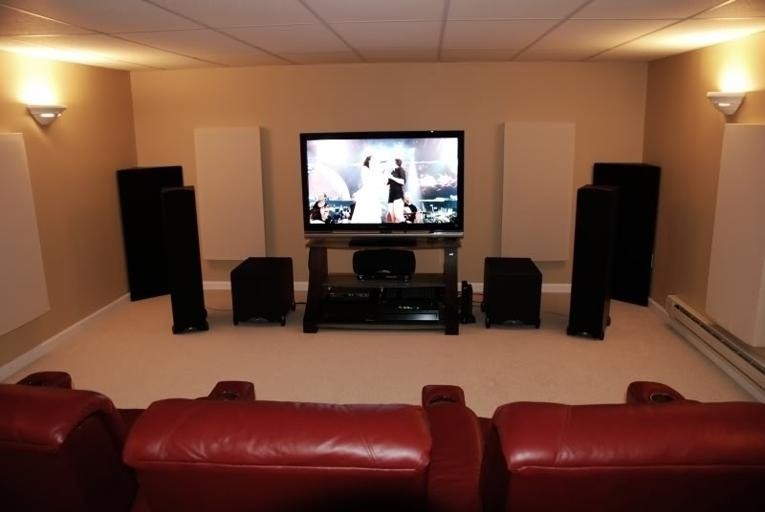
[229,256,295,326]
[115,165,184,304]
[351,249,417,278]
[567,185,615,340]
[161,185,205,334]
[480,255,542,329]
[593,161,661,306]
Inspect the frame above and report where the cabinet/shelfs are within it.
[302,239,461,335]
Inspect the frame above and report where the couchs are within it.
[1,369,762,511]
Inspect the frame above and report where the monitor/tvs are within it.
[299,128,464,246]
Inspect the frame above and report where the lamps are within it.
[26,105,67,128]
[706,92,745,116]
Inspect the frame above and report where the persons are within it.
[310,192,329,224]
[383,156,407,221]
[352,155,383,223]
